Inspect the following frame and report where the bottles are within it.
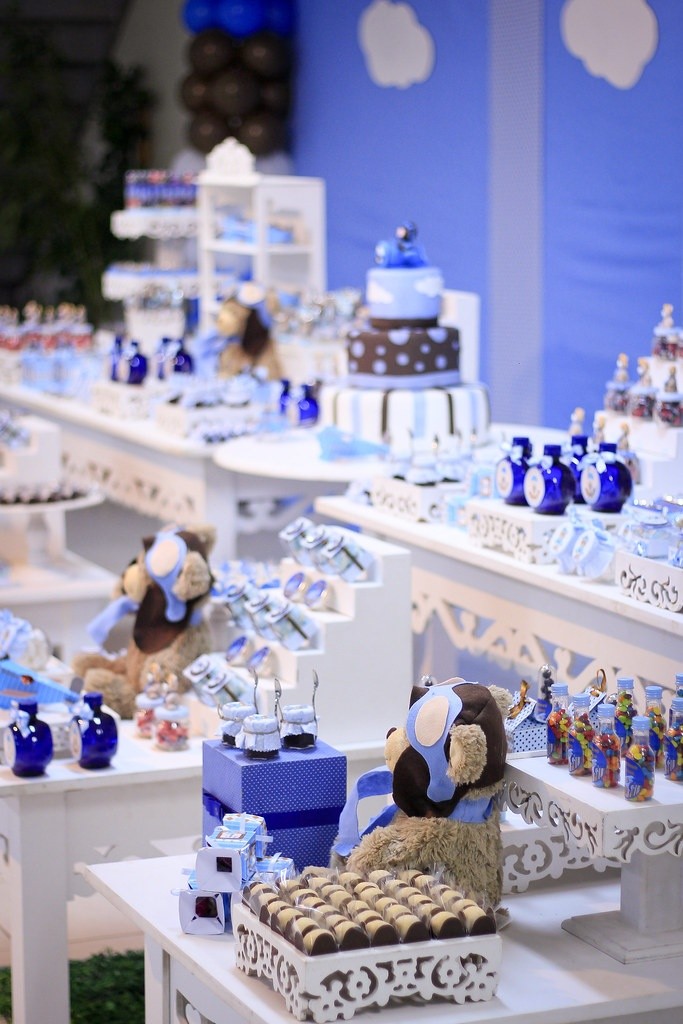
[568,693,592,775]
[615,677,638,757]
[4,699,54,776]
[0,302,94,393]
[495,436,530,505]
[669,673,683,727]
[299,383,319,424]
[548,683,567,764]
[526,445,576,513]
[570,433,590,505]
[579,443,632,514]
[626,716,654,802]
[644,686,667,772]
[279,378,290,414]
[592,704,619,788]
[116,342,146,384]
[70,695,119,767]
[664,699,683,781]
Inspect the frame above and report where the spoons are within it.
[274,679,282,732]
[248,666,258,713]
[311,669,319,722]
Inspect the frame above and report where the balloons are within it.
[1,0,163,326]
[175,0,301,162]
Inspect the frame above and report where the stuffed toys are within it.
[68,523,220,722]
[209,281,287,381]
[342,677,510,929]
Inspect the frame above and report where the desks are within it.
[86,807,683,1024]
[0,375,317,566]
[0,531,123,652]
[311,496,682,721]
[1,655,411,1024]
[212,423,573,484]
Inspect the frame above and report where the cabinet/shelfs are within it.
[103,173,325,348]
